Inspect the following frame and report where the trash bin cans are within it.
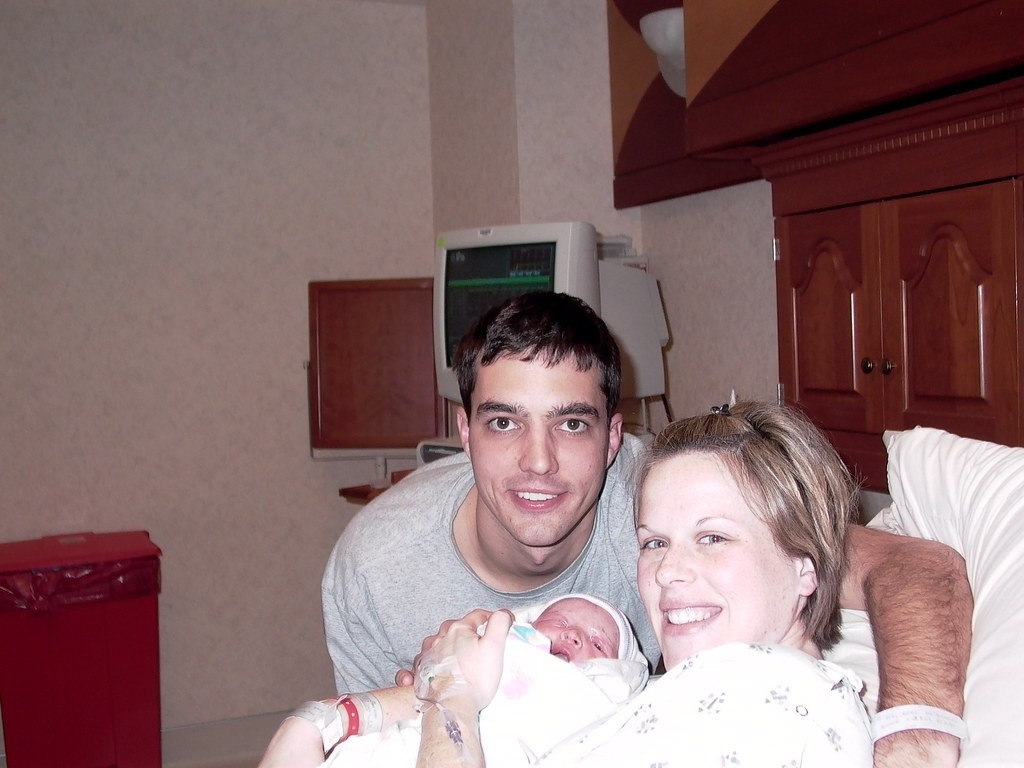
[0,525,165,768]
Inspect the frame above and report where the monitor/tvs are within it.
[435,220,668,404]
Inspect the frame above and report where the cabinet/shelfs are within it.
[606,0,1024,498]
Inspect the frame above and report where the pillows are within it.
[823,427,1024,767]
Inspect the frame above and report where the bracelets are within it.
[331,696,359,743]
[352,691,382,736]
[873,704,968,748]
[279,700,343,752]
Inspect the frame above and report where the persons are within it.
[320,290,974,768]
[256,402,875,768]
